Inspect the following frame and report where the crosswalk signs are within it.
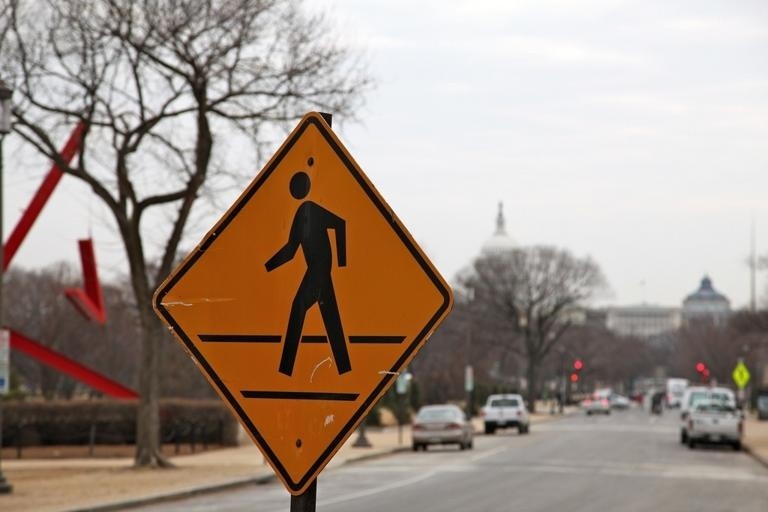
[150,111,456,498]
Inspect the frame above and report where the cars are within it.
[583,377,745,450]
[480,393,532,435]
[411,403,472,451]
